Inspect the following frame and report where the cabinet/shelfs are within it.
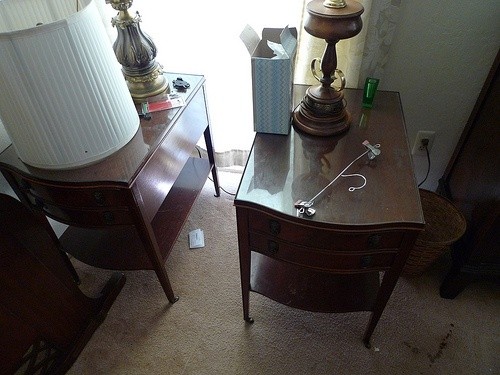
[233,85,427,349]
[1,70,221,303]
[435,48,500,300]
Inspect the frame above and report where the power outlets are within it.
[412,130,436,156]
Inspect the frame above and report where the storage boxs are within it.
[240,24,298,135]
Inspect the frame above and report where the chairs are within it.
[0,193,126,375]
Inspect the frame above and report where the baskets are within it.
[400,188,467,277]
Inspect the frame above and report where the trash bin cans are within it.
[400,187,468,279]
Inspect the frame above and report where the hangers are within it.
[295,139,381,216]
